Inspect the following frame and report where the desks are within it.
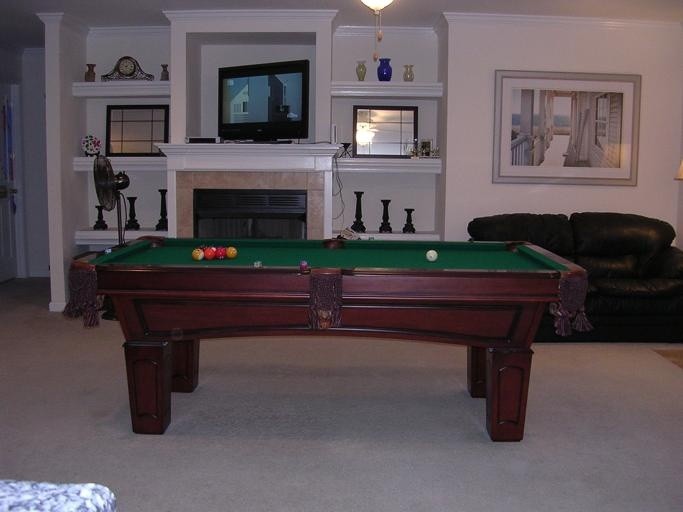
[63,236,594,434]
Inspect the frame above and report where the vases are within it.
[377,59,392,81]
[404,65,414,81]
[356,63,366,81]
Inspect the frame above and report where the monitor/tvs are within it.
[217,59,310,144]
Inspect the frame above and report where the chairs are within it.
[570,212,683,342]
[467,213,577,341]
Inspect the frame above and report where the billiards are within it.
[191,244,237,259]
[427,250,438,261]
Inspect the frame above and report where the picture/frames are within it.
[492,70,641,186]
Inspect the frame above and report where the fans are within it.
[93,156,130,320]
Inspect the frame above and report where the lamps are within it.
[361,0,399,61]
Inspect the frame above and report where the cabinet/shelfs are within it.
[330,29,442,240]
[72,26,171,245]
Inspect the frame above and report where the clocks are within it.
[101,56,154,81]
